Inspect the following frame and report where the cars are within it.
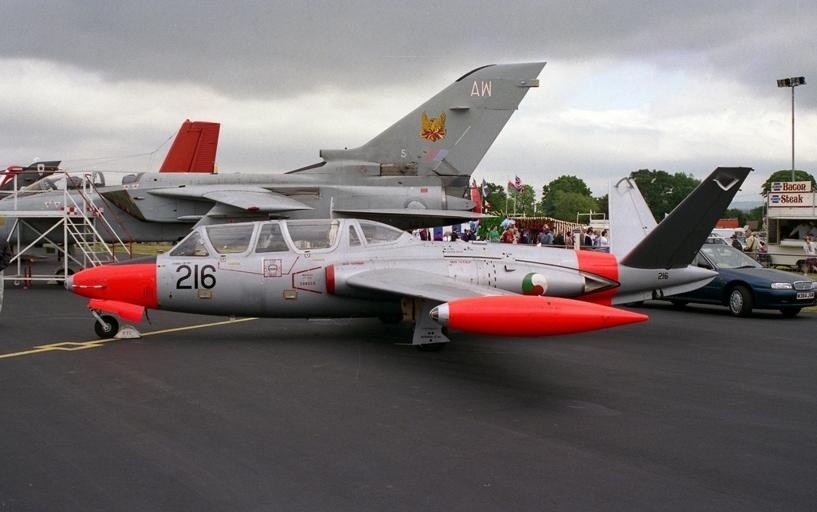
[585,232,816,317]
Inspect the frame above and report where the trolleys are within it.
[0,169,134,292]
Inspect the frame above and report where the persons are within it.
[417,217,606,250]
[728,228,816,277]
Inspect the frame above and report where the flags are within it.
[505,175,524,193]
[481,179,489,197]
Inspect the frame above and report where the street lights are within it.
[777,75,807,182]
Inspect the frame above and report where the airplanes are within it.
[0,61,549,287]
[62,164,755,348]
